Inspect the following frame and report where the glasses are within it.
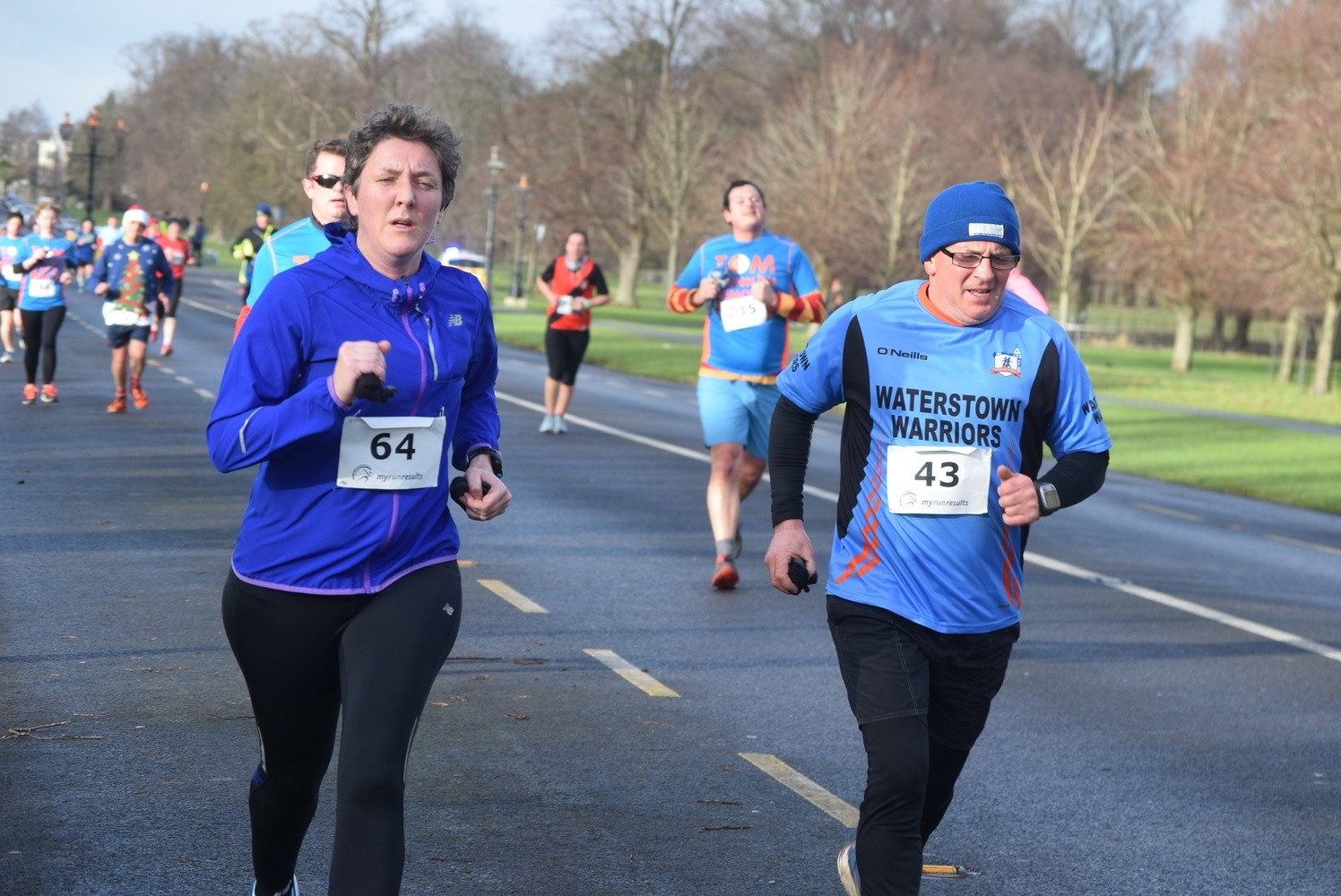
[940,246,1020,271]
[308,174,345,188]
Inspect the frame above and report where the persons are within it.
[208,104,512,896]
[765,182,1112,896]
[537,228,611,431]
[233,136,350,340]
[668,182,832,589]
[229,203,278,303]
[0,201,208,412]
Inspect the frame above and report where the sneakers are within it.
[712,555,740,590]
[107,397,127,413]
[130,384,149,409]
[40,383,58,402]
[22,383,39,404]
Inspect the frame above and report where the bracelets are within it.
[583,299,590,308]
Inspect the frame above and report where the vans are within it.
[436,247,487,294]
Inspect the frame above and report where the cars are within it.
[4,193,38,226]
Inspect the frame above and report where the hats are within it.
[919,180,1020,268]
[122,205,151,228]
[256,202,272,218]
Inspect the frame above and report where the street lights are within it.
[513,173,529,298]
[57,110,127,224]
[484,145,505,310]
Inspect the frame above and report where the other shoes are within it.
[149,323,159,341]
[15,326,29,350]
[554,415,570,434]
[540,415,554,434]
[837,842,861,896]
[251,875,299,896]
[0,350,15,363]
[160,345,173,355]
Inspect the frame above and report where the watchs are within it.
[1034,478,1059,516]
[464,446,504,479]
